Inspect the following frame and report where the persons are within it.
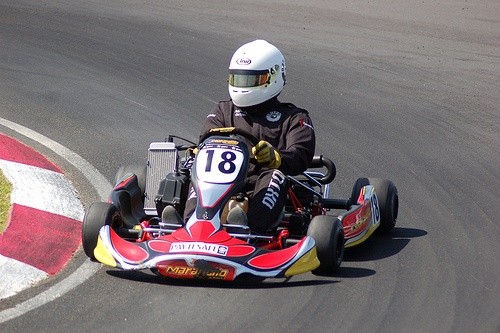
[154,40,316,246]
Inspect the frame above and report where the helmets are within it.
[228,40,286,107]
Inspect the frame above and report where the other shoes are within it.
[162,205,183,229]
[225,205,247,233]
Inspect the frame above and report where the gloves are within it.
[252,141,281,169]
